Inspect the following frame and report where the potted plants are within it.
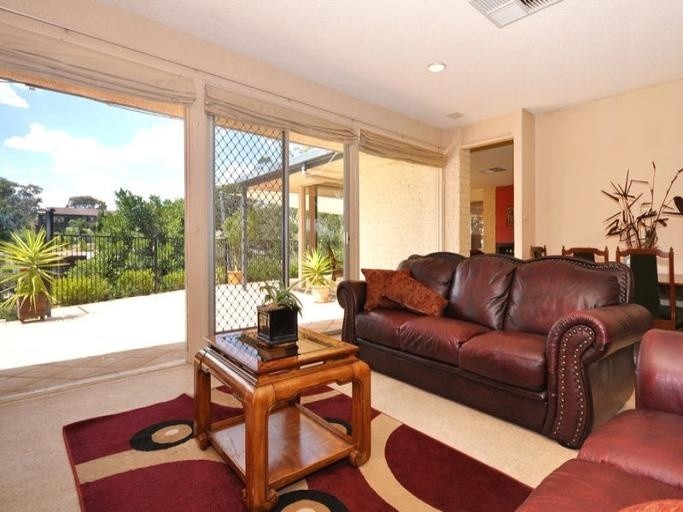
[0,224,73,324]
[286,245,339,303]
[256,278,304,348]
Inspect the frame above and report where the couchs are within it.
[337,251,655,451]
[514,329,682,511]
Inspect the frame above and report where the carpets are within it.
[62,380,534,512]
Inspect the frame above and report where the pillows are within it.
[355,265,450,319]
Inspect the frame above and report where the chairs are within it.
[528,240,678,330]
